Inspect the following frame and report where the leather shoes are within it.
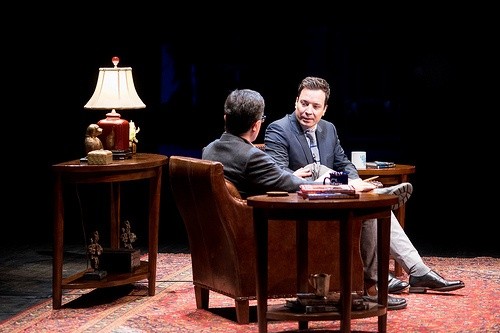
[388,277,409,293]
[362,290,407,310]
[409,270,465,292]
[373,182,413,211]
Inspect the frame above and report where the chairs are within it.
[169,144,365,324]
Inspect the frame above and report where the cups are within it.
[352,152,366,170]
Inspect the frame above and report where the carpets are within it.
[0,252,500,333]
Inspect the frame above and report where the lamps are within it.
[83,56,147,161]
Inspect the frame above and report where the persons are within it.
[201,89,407,310]
[121,222,137,251]
[264,77,465,292]
[87,232,103,271]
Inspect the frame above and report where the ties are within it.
[305,130,320,164]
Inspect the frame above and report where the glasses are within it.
[254,116,266,124]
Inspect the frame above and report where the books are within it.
[366,161,396,169]
[297,184,360,200]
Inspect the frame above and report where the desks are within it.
[50,154,169,310]
[357,163,416,277]
[247,195,399,333]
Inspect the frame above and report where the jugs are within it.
[308,273,333,298]
